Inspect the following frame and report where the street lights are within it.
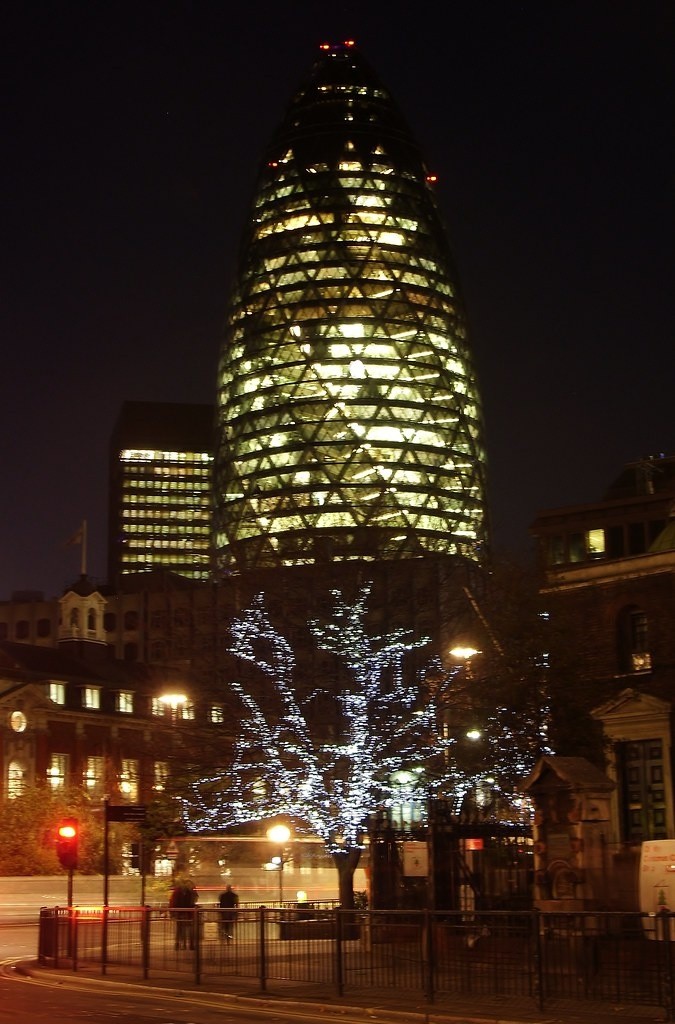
[265,824,291,907]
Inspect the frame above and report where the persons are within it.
[182,880,200,950]
[168,875,190,950]
[218,884,240,939]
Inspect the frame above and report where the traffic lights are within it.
[55,817,79,871]
